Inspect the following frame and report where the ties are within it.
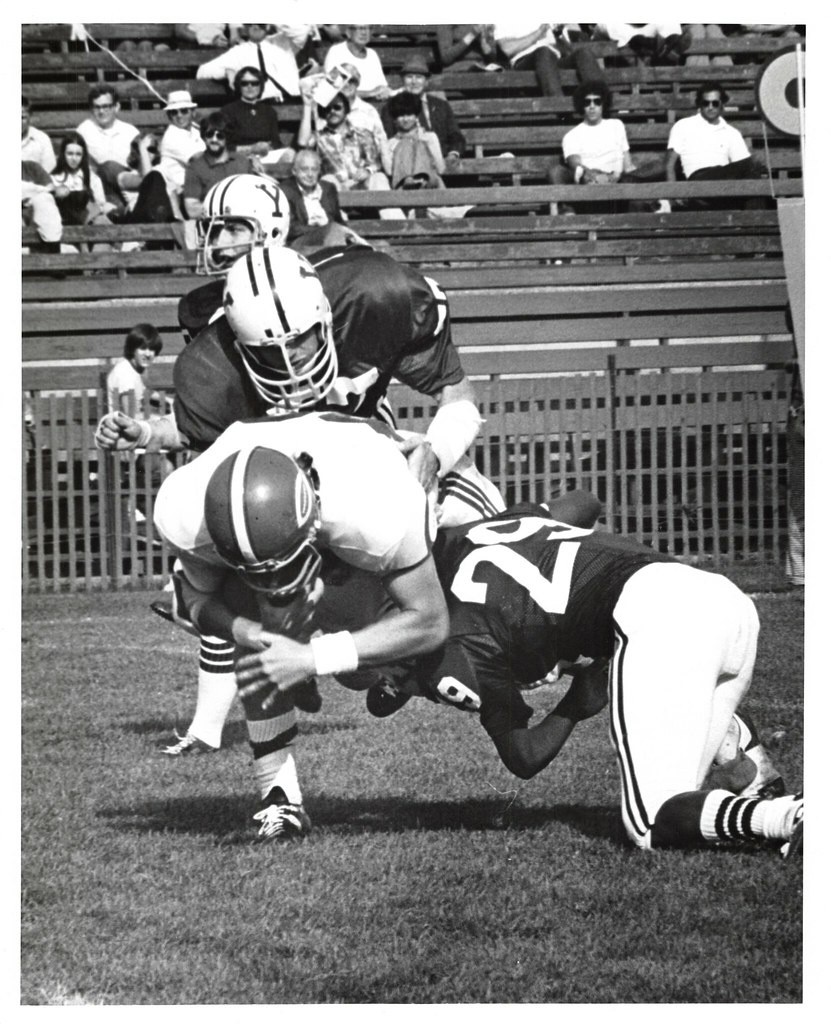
[257,43,267,80]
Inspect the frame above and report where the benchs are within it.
[21,25,806,469]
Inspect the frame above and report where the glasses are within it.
[320,104,345,113]
[146,146,157,153]
[240,81,261,87]
[701,100,721,106]
[169,108,192,116]
[582,98,602,106]
[206,130,225,139]
[91,104,115,112]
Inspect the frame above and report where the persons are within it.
[23,395,37,458]
[665,83,763,211]
[108,318,172,525]
[90,173,802,857]
[547,79,671,216]
[22,24,800,252]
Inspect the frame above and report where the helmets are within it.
[205,446,324,599]
[195,173,290,276]
[224,246,339,409]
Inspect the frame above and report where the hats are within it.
[163,91,197,110]
[399,55,431,79]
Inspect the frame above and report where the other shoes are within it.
[645,34,669,64]
[662,32,691,67]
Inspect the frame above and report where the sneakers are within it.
[160,728,220,757]
[253,785,311,849]
[782,795,803,865]
[709,713,783,798]
